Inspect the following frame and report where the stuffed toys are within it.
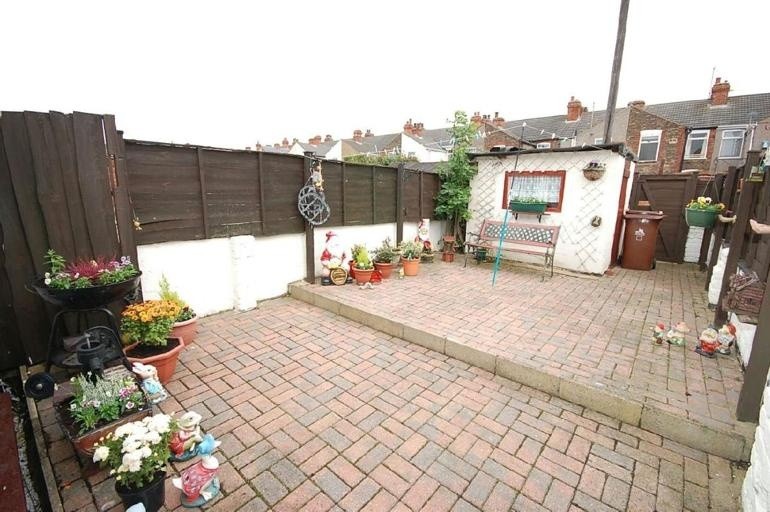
[133,361,167,404]
[171,412,202,461]
[651,320,736,358]
[171,434,222,508]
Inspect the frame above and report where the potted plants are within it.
[347,243,375,286]
[401,240,423,276]
[158,270,198,347]
[368,236,396,280]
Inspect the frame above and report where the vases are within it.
[115,470,167,512]
[56,381,154,464]
[23,271,146,310]
[122,335,185,386]
[684,207,721,229]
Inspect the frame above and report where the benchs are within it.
[463,219,561,282]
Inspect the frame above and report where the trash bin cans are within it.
[622,209,665,271]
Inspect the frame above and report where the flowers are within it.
[41,248,140,290]
[686,196,725,211]
[91,412,180,490]
[119,298,182,348]
[53,370,146,438]
